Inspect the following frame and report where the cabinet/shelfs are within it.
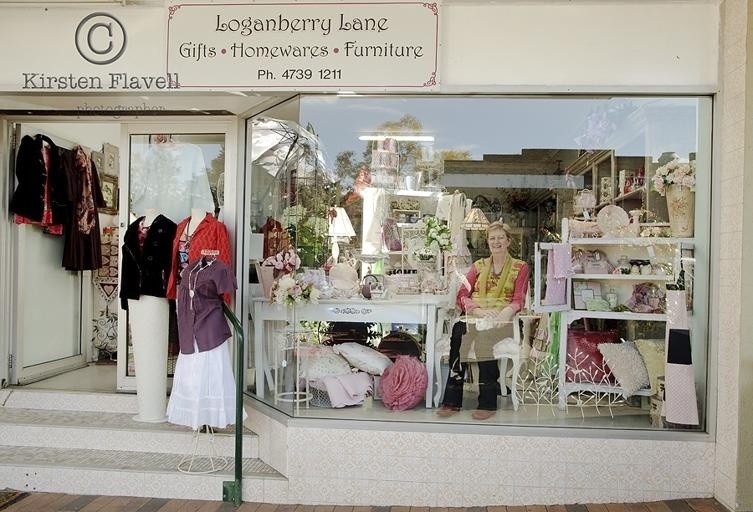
[556,236,678,419]
[571,132,651,222]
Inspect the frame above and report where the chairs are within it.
[434,265,522,410]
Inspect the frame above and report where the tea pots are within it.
[361,275,379,298]
[370,282,388,300]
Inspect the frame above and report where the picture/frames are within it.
[100,173,118,214]
[94,153,102,170]
[104,142,120,175]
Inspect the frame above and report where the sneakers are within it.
[435,404,461,417]
[472,408,497,420]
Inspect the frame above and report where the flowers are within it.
[653,150,696,194]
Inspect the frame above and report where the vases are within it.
[666,182,693,238]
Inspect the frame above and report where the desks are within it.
[252,282,440,410]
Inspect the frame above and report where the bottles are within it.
[617,255,630,273]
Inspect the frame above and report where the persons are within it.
[118,205,175,310]
[432,219,528,420]
[168,207,231,307]
[173,253,235,438]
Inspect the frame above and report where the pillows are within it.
[566,327,666,400]
[333,342,393,378]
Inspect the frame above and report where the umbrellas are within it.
[249,117,340,205]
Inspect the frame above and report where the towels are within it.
[544,245,571,303]
